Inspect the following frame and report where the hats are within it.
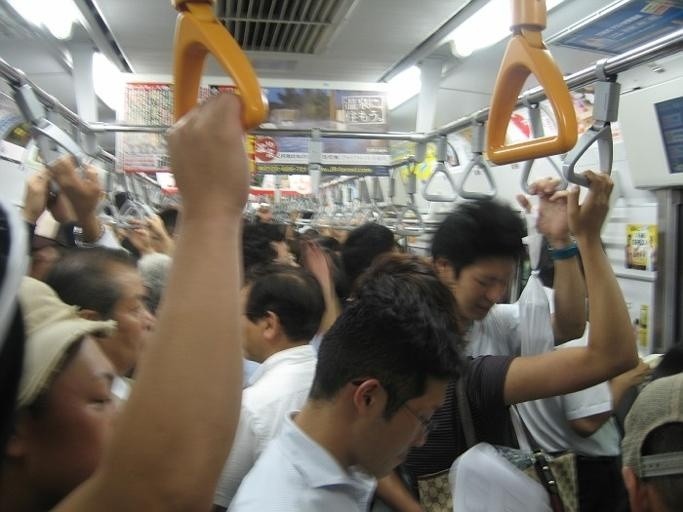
[620,373,683,478]
[14,277,118,411]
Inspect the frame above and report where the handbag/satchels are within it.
[417,354,580,512]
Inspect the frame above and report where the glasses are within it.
[351,379,437,436]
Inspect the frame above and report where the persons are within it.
[0,91,682,512]
